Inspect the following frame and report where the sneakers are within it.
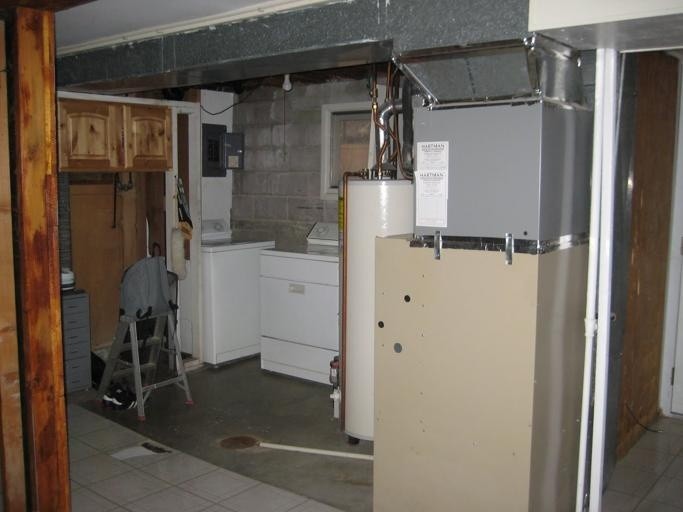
[101,385,149,411]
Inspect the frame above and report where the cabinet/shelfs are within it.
[62,294,92,393]
[56,90,173,172]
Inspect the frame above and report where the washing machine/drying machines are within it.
[258,222,340,385]
[200,217,275,369]
